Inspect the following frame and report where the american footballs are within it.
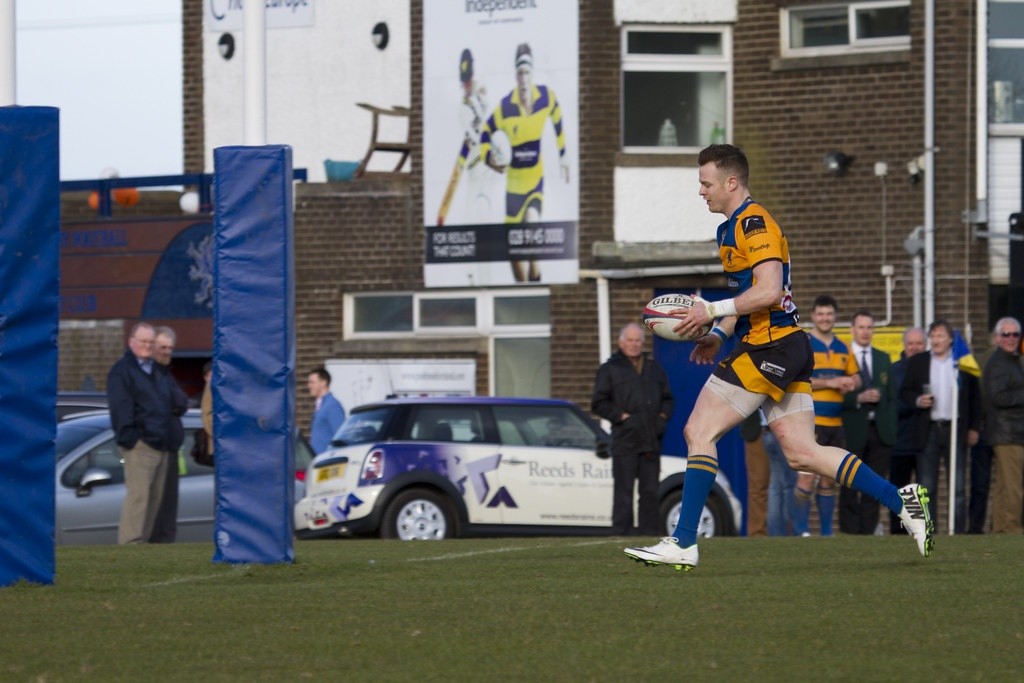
[642,292,714,342]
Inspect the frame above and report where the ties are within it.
[860,350,872,388]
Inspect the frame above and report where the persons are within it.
[439,43,572,282]
[623,143,936,572]
[588,294,1024,536]
[108,321,214,545]
[309,368,346,456]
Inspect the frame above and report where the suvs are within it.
[295,392,743,542]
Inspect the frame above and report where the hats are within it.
[516,43,534,67]
[460,49,473,82]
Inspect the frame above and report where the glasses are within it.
[998,329,1021,338]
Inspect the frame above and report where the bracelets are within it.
[709,325,729,344]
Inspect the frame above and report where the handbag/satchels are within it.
[190,426,217,466]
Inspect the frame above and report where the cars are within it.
[54,411,305,545]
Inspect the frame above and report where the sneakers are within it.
[895,484,936,559]
[623,539,700,571]
